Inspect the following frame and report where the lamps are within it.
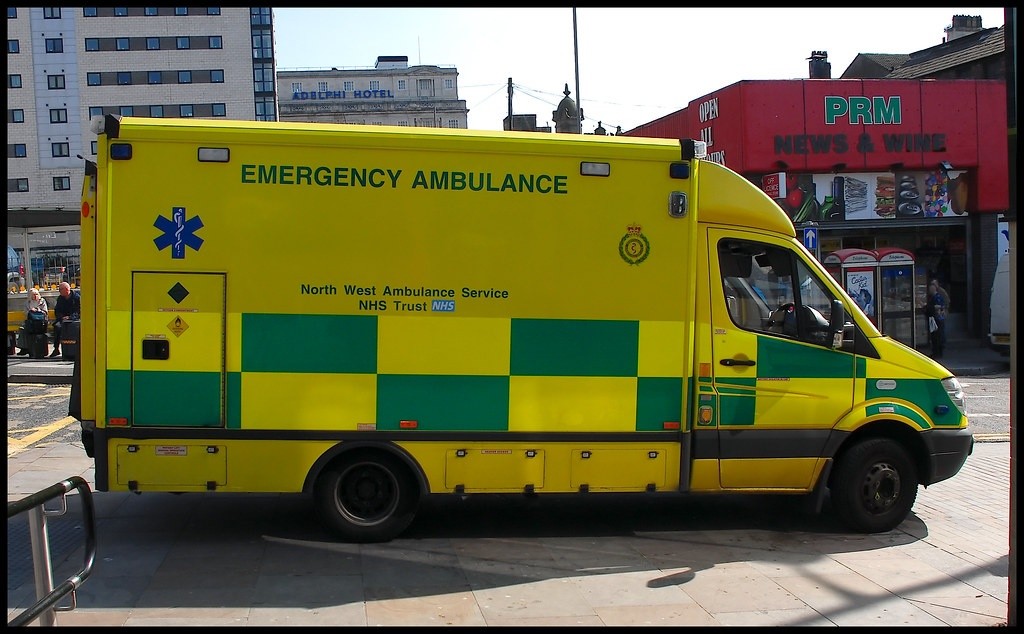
[890,163,903,173]
[940,161,953,172]
[833,164,846,174]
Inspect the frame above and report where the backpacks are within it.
[27,311,45,334]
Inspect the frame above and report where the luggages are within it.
[62,318,80,361]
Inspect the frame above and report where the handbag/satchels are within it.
[929,316,938,333]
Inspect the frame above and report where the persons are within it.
[49,282,81,358]
[848,289,874,315]
[924,278,950,358]
[16,288,48,355]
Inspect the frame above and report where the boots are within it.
[8,347,15,355]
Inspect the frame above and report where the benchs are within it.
[8,310,56,355]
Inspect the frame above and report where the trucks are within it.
[44,266,64,284]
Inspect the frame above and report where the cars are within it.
[7,272,24,292]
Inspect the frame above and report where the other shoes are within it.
[50,350,60,357]
[17,349,29,355]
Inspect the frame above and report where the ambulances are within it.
[72,116,974,543]
